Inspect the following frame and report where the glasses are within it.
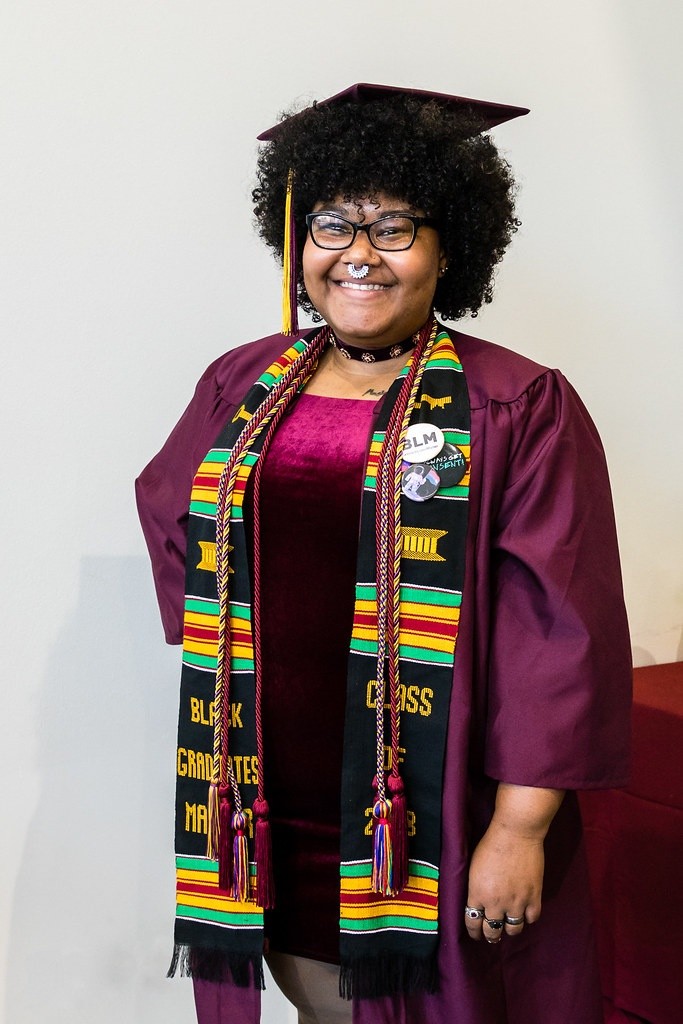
[303,209,434,252]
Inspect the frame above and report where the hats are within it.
[256,81,532,337]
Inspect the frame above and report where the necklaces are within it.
[327,314,431,362]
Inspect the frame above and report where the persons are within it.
[130,80,637,1024]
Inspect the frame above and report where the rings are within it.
[464,905,485,919]
[483,914,504,929]
[505,914,523,925]
